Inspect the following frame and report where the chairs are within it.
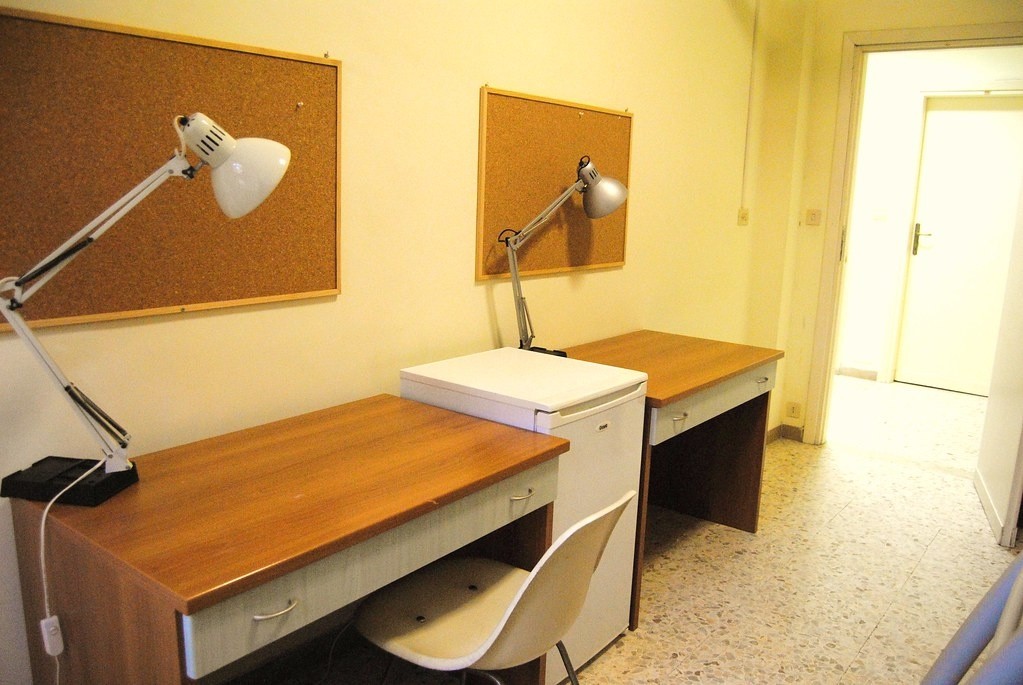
[324,490,634,685]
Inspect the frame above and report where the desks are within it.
[561,326,784,635]
[0,394,569,685]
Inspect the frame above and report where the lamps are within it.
[0,109,292,508]
[504,155,628,358]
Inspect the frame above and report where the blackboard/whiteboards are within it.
[475,86,631,277]
[0,4,341,334]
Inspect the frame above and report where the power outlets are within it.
[786,401,801,418]
